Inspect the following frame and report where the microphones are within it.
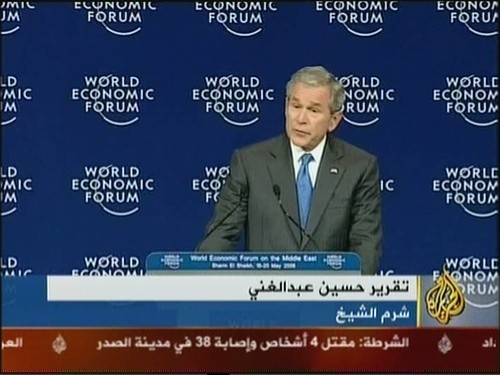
[273,184,327,251]
[195,184,248,251]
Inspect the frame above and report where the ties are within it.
[296,154,314,231]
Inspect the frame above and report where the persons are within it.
[195,66,382,275]
